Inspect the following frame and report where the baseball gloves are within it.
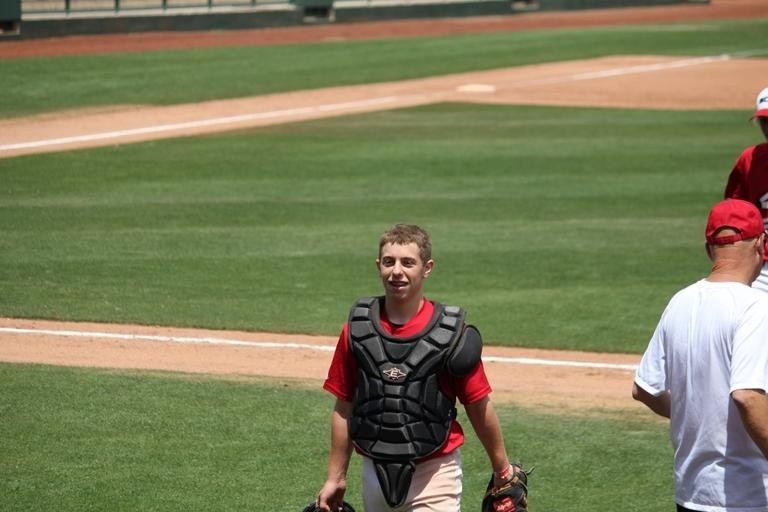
[480,461,528,511]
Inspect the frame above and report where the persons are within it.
[313,223,518,511]
[722,85,768,296]
[629,197,768,511]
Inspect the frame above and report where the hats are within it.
[749,88,768,125]
[704,197,767,249]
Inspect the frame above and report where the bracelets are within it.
[494,463,512,479]
